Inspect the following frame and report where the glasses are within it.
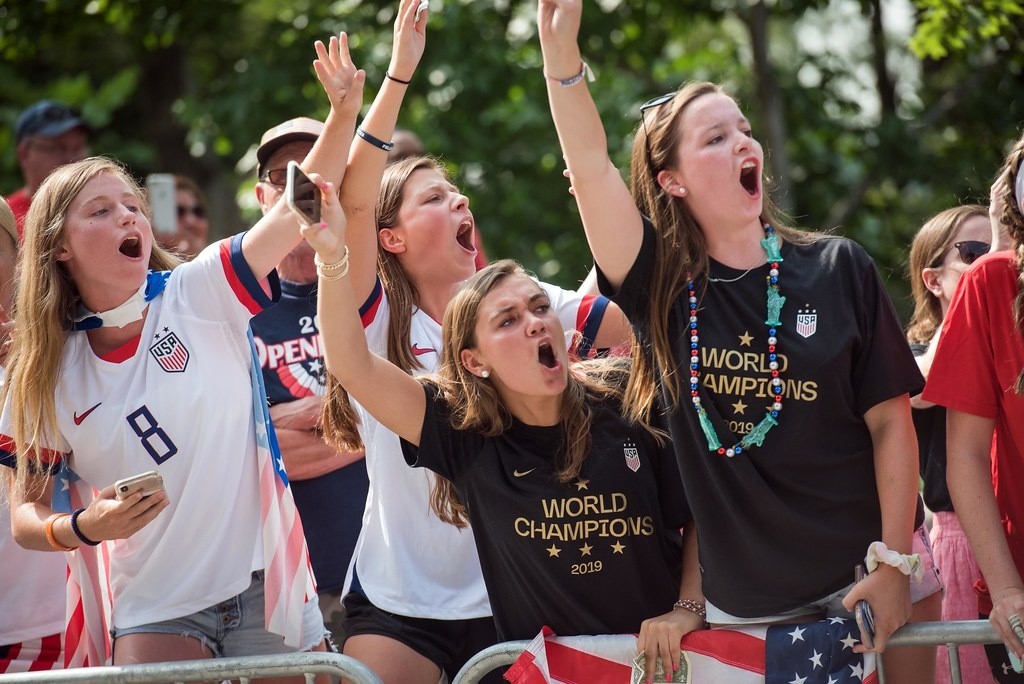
[260,169,300,185]
[932,241,991,265]
[640,94,675,150]
[177,204,205,219]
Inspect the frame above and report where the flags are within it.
[502,616,879,684]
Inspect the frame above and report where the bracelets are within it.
[356,125,394,151]
[386,72,411,84]
[865,541,923,579]
[317,260,349,281]
[673,599,707,626]
[543,58,596,87]
[314,245,349,271]
[45,512,80,552]
[71,508,102,546]
[904,207,997,684]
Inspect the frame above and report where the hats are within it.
[257,117,325,179]
[15,101,87,145]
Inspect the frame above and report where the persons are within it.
[922,132,1024,684]
[0,3,641,684]
[300,172,708,684]
[536,0,947,684]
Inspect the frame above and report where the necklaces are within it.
[686,214,785,457]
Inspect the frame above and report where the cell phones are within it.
[286,160,322,225]
[855,564,876,650]
[114,469,168,506]
[146,173,177,236]
